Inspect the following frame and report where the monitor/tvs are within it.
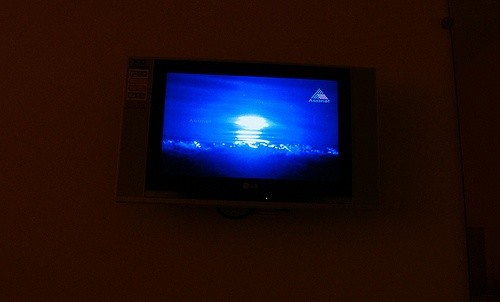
[112,56,381,214]
